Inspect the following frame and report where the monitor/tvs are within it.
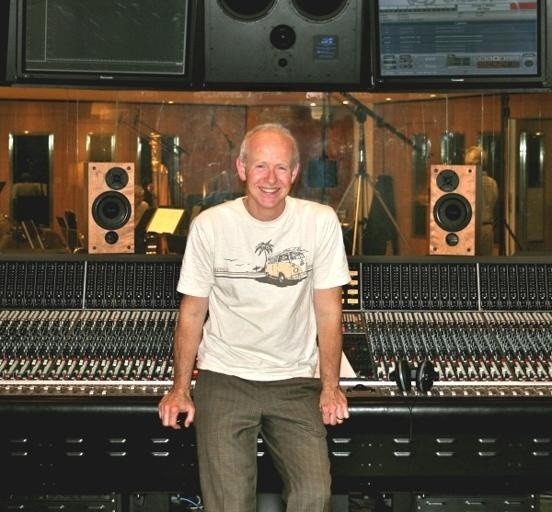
[4,0,199,90]
[370,0,552,91]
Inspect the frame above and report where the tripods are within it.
[335,173,410,256]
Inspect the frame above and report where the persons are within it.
[461,145,498,255]
[159,123,351,512]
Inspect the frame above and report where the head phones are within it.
[389,360,439,393]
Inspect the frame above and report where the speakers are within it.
[85,132,116,162]
[441,133,465,163]
[137,135,180,223]
[8,134,55,243]
[203,0,371,92]
[526,135,544,188]
[429,165,482,257]
[83,162,135,254]
[478,131,527,252]
